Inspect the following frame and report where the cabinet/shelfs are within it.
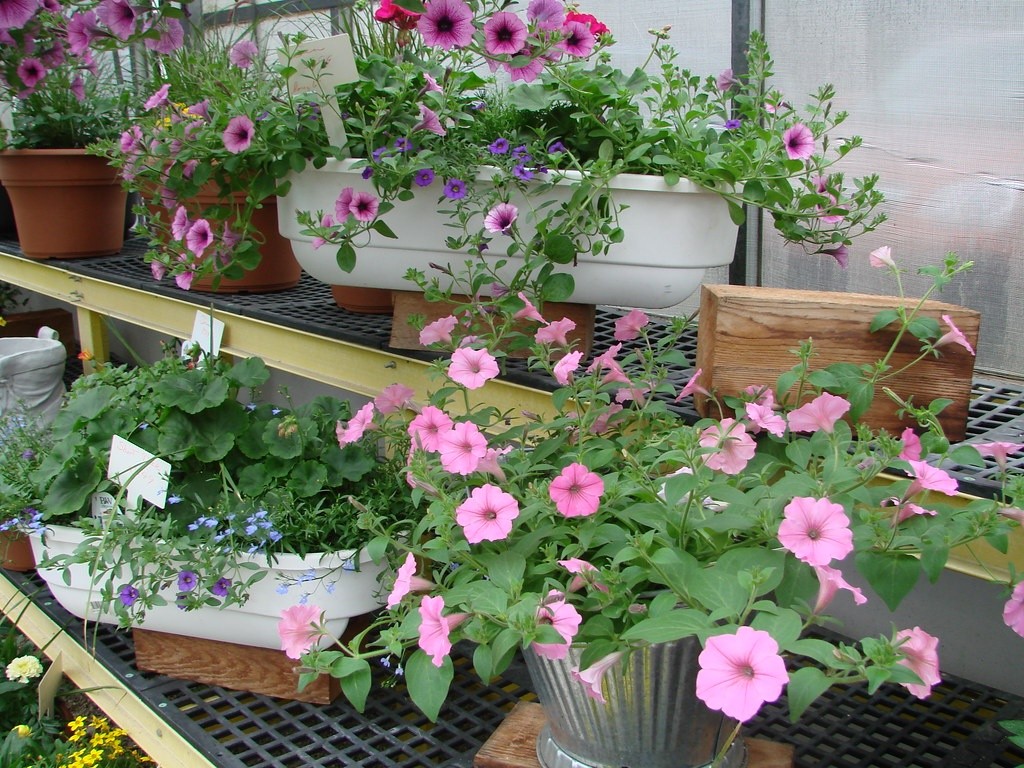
[0,246,1024,768]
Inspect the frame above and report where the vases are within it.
[135,155,301,294]
[273,152,746,311]
[0,520,37,572]
[28,521,408,653]
[0,150,127,260]
[517,635,749,768]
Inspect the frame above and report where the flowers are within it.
[0,0,1024,768]
